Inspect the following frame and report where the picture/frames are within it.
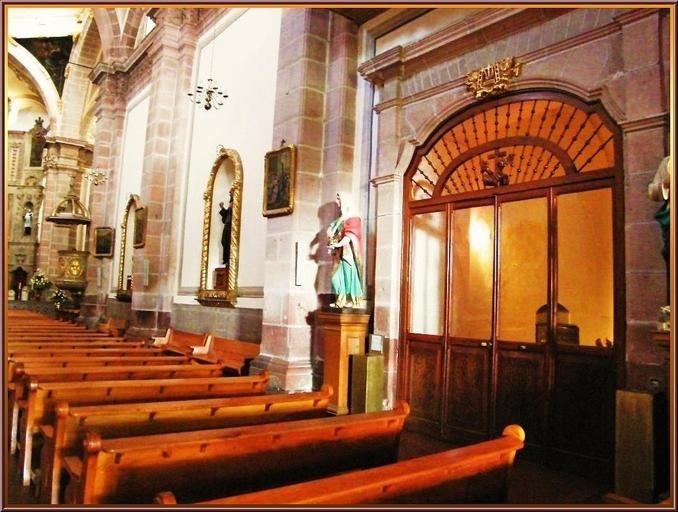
[93,226,115,258]
[134,206,147,248]
[261,144,297,216]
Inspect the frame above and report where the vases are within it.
[35,288,42,299]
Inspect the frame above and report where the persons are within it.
[326,216,364,308]
[23,208,32,235]
[219,187,234,269]
[648,156,670,305]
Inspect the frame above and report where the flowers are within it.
[30,270,50,290]
[48,288,68,308]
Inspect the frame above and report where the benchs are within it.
[6,301,526,504]
